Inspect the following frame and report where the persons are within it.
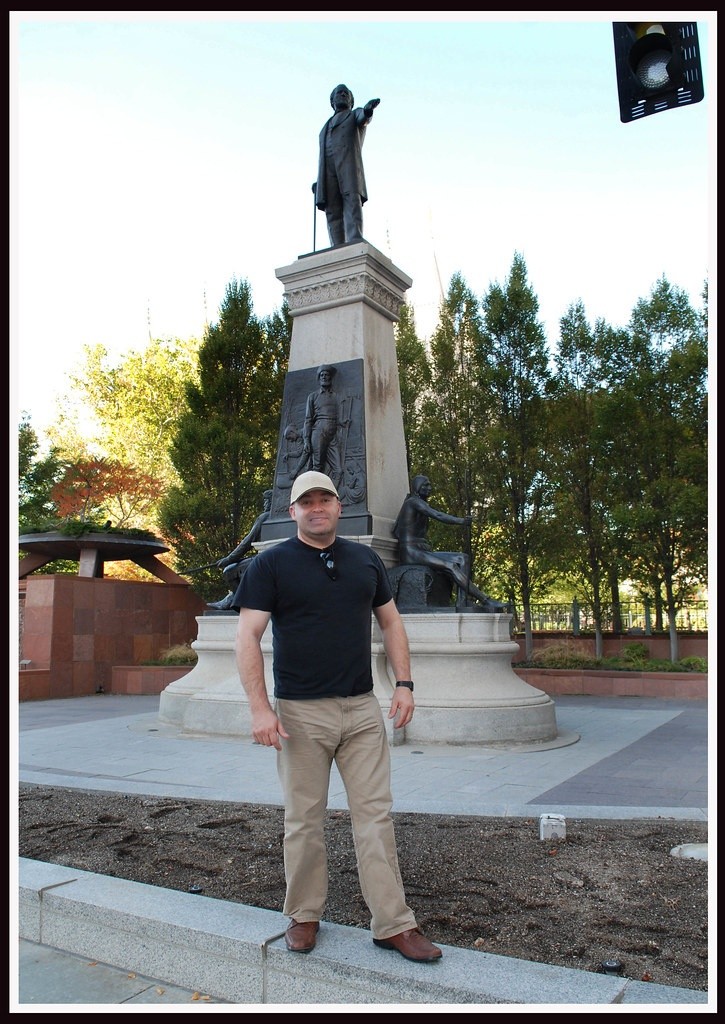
[311,84,381,246]
[206,490,274,610]
[302,365,352,493]
[282,425,313,479]
[338,460,367,504]
[230,470,443,963]
[391,474,510,609]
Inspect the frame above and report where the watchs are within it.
[395,681,414,692]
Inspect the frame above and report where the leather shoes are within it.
[372,927,444,964]
[285,917,320,953]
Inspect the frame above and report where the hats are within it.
[290,471,340,504]
